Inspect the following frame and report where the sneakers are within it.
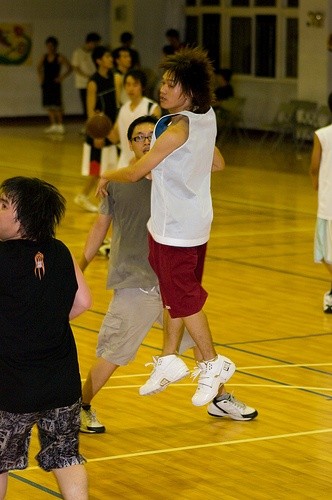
[190,354,236,406]
[78,404,105,433]
[138,354,191,396]
[208,393,258,421]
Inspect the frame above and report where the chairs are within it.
[215,96,249,149]
[255,100,332,160]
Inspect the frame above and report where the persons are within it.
[71,32,102,133]
[100,66,162,258]
[311,92,332,312]
[37,36,74,133]
[0,176,92,500]
[94,48,235,405]
[75,45,132,211]
[111,31,141,69]
[162,28,184,59]
[79,114,258,432]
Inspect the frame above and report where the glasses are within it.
[130,135,152,143]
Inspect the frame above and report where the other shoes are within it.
[44,125,66,136]
[96,238,111,258]
[74,194,99,213]
[323,291,332,312]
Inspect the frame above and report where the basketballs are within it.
[88,115,112,139]
